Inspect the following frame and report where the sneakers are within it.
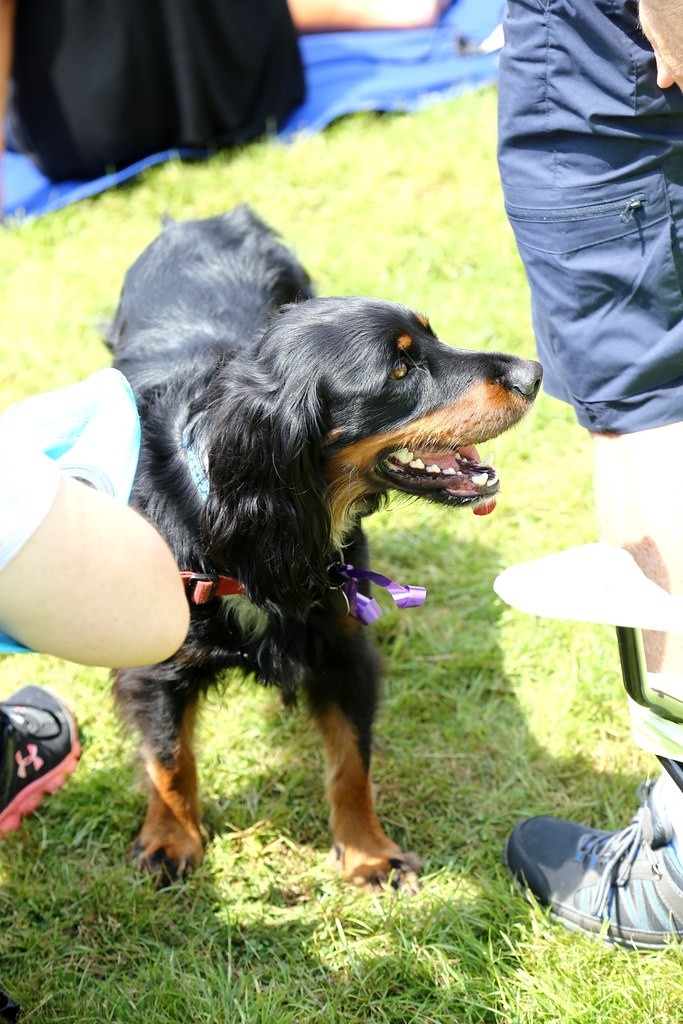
[0,684,84,843]
[508,812,683,953]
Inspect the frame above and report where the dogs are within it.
[106,203,545,897]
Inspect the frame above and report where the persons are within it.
[1,0,454,224]
[0,369,189,836]
[498,0,683,958]
